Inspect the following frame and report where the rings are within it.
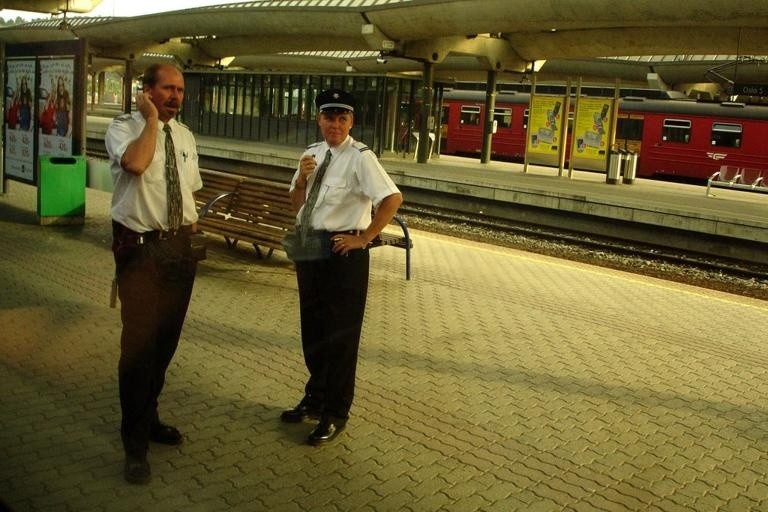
[342,243,344,247]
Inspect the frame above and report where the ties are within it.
[163,123,183,231]
[300,150,331,246]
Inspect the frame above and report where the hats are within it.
[316,89,355,113]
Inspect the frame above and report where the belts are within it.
[327,230,361,235]
[123,230,159,245]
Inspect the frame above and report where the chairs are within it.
[706,164,768,197]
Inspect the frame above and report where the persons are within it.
[281,89,404,443]
[5,76,71,136]
[105,61,204,485]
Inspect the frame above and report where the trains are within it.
[396,86,768,194]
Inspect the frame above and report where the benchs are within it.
[189,168,416,280]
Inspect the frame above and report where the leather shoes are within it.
[150,419,182,445]
[125,448,151,483]
[280,396,349,446]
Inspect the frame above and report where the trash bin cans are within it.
[38,154,87,226]
[606,148,639,185]
